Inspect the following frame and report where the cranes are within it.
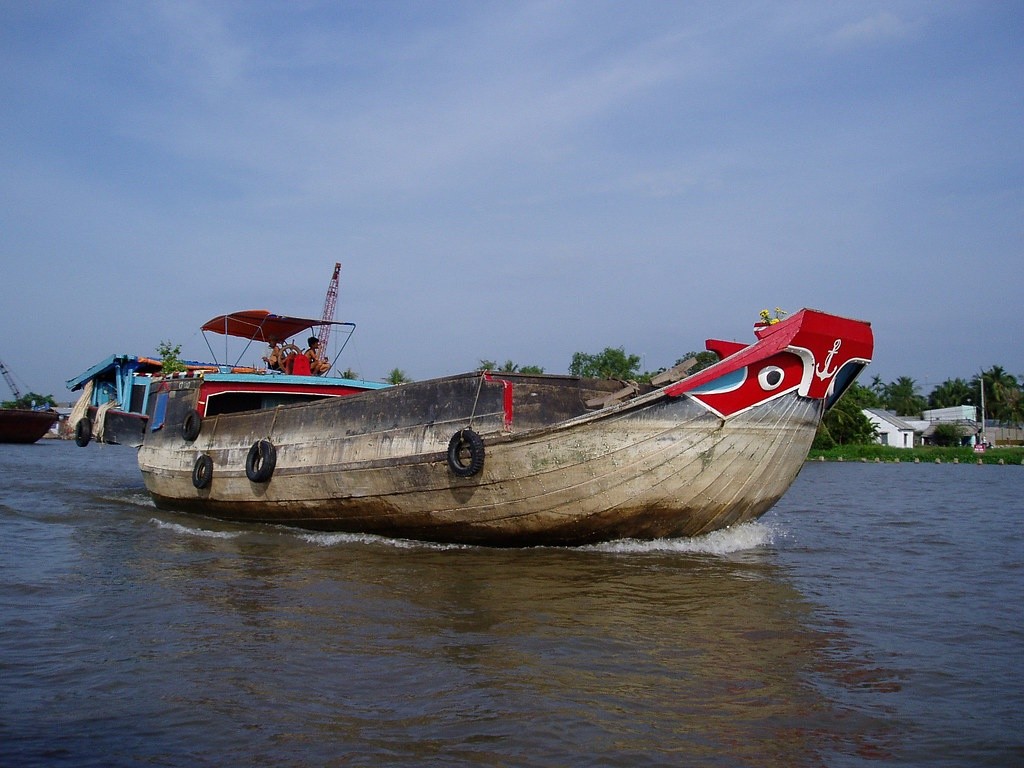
[0,359,24,404]
[309,263,347,376]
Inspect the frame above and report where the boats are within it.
[0,401,58,446]
[64,310,876,552]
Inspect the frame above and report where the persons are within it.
[260,339,285,371]
[303,336,331,376]
